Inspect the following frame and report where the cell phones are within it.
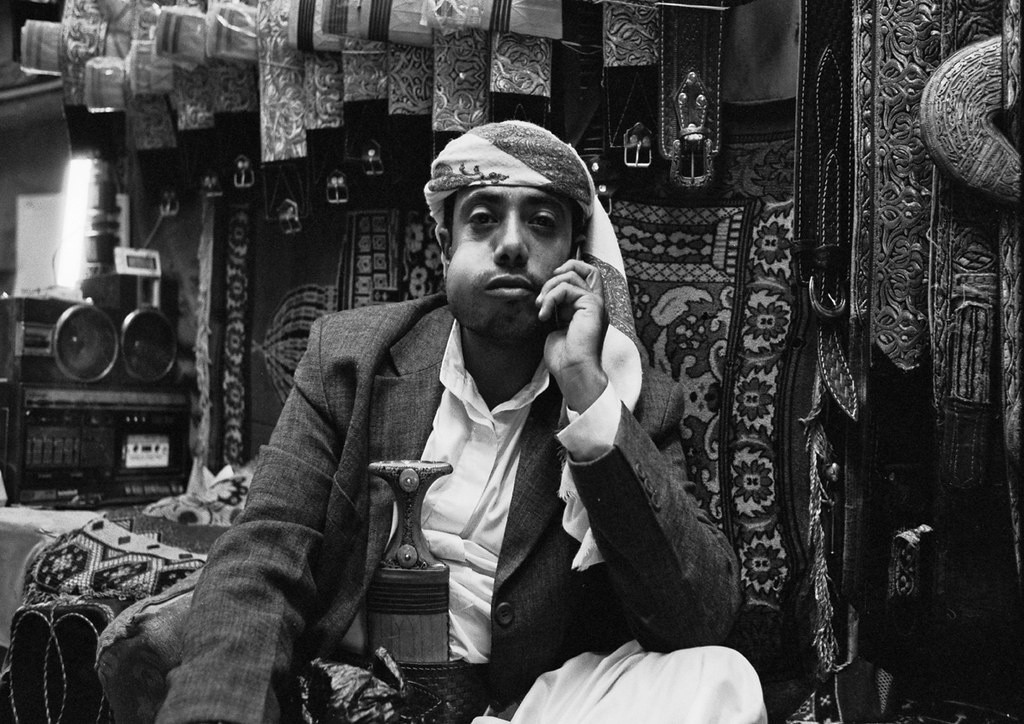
[554,245,584,330]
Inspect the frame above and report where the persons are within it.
[155,120,767,724]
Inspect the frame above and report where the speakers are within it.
[0,298,182,394]
